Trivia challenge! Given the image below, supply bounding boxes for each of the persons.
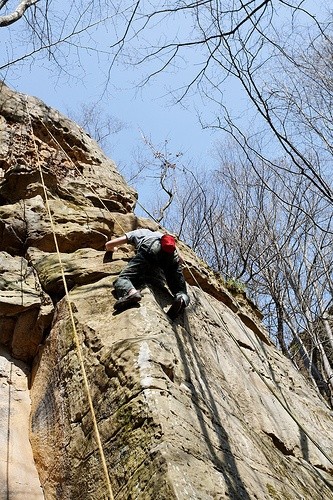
[105,228,190,321]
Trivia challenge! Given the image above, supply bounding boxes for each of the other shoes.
[114,292,141,309]
[167,297,183,320]
